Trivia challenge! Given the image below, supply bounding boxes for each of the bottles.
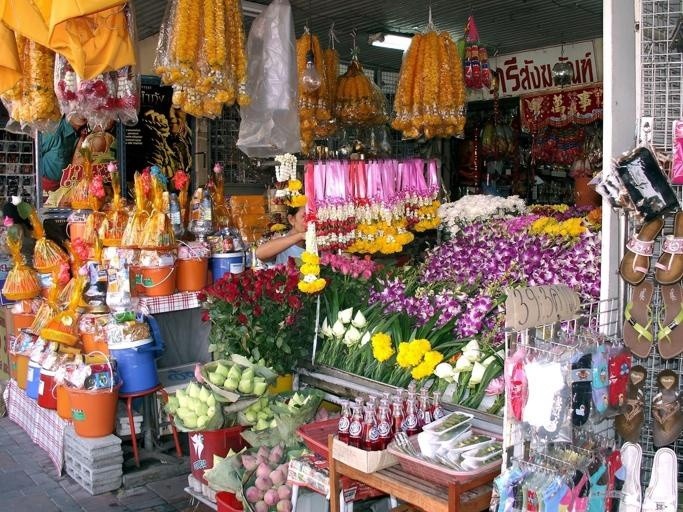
[171,194,181,235]
[337,382,442,451]
[203,191,213,234]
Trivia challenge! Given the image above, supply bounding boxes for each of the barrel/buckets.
[8,333,22,379]
[15,352,30,390]
[140,266,177,296]
[25,360,41,400]
[11,312,35,335]
[127,265,145,294]
[82,334,108,357]
[37,368,57,410]
[63,350,123,438]
[212,252,244,283]
[177,257,209,291]
[51,382,71,420]
[65,222,88,242]
[106,337,161,393]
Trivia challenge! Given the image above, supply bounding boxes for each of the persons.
[255,207,307,265]
[142,105,192,180]
[41,113,86,206]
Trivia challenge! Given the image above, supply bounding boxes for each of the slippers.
[612,363,648,445]
[654,209,683,287]
[639,447,679,512]
[616,441,644,512]
[621,280,655,359]
[649,368,683,448]
[656,283,683,362]
[618,216,666,286]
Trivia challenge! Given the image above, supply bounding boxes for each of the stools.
[118,385,183,468]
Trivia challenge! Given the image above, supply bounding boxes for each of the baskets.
[39,328,78,347]
[386,425,504,488]
[32,253,71,274]
[2,286,41,301]
[294,410,385,503]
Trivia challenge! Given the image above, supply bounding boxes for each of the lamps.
[300,0,322,91]
[552,42,574,86]
[368,32,414,51]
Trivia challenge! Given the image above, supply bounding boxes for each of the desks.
[116,294,202,316]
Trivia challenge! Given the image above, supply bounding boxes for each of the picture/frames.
[33,119,127,219]
